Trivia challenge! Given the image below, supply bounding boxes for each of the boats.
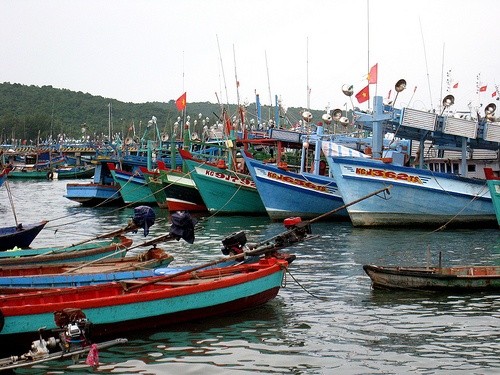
[363,239,499,298]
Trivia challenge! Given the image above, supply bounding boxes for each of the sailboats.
[0,0,500,375]
